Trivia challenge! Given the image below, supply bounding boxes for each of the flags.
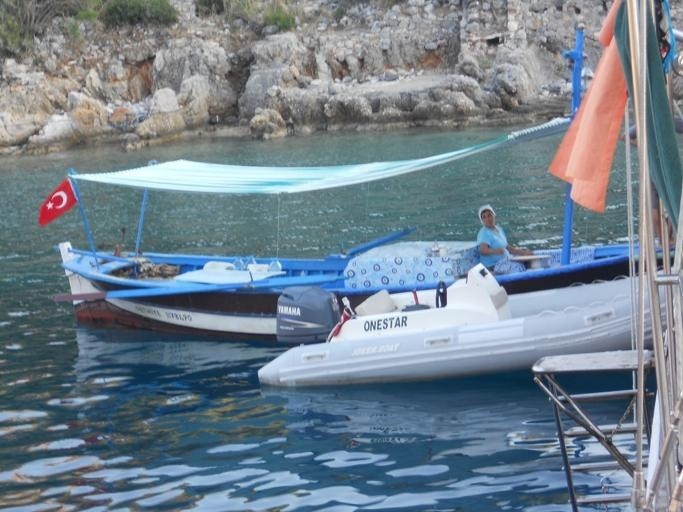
[38,176,78,228]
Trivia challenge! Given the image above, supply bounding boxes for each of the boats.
[57,23,680,342]
[257,262,676,392]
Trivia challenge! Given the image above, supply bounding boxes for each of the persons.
[475,204,533,274]
[617,103,676,247]
